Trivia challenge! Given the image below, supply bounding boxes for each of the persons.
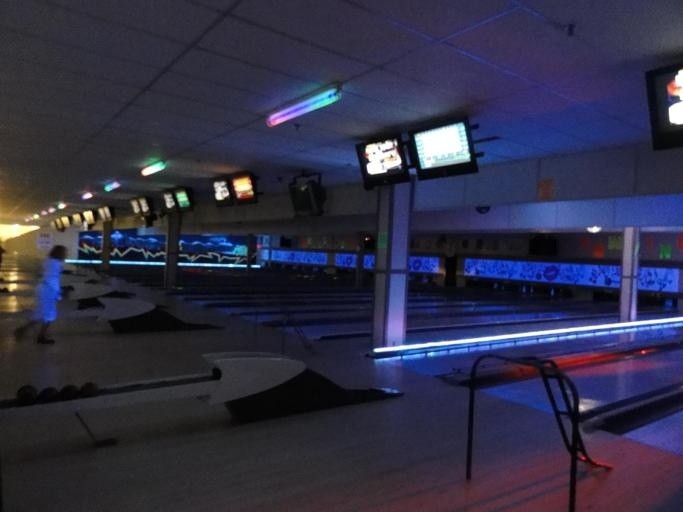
[14,245,65,344]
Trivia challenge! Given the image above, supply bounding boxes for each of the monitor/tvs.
[288,180,327,215]
[355,132,411,190]
[49,170,259,231]
[408,116,479,181]
[645,62,683,151]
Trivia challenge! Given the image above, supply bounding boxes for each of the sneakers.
[14,327,56,345]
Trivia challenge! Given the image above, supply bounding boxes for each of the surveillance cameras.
[475,205,491,214]
